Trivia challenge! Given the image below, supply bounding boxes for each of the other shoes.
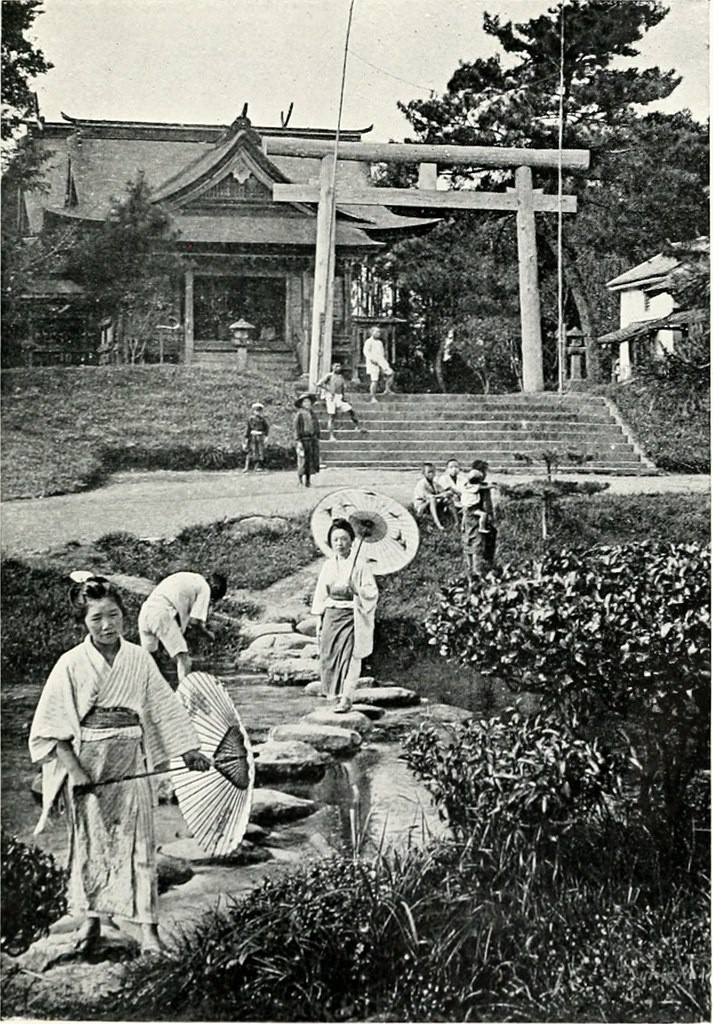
[71,926,101,953]
[333,704,352,713]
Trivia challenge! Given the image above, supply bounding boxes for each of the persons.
[363,325,396,402]
[460,458,501,572]
[316,359,367,442]
[134,568,228,684]
[439,457,469,508]
[292,393,321,487]
[309,515,380,714]
[25,574,212,955]
[241,403,268,472]
[411,462,460,530]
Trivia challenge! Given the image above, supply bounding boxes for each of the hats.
[294,393,317,408]
[252,403,264,410]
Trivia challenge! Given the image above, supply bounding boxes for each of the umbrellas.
[71,669,260,855]
[309,486,421,585]
